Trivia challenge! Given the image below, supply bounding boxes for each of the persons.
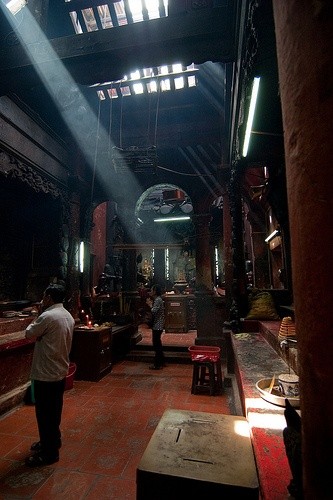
[146,285,165,370]
[24,283,76,466]
[59,248,67,280]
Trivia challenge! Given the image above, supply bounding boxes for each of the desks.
[136,409,259,500]
[112,323,132,335]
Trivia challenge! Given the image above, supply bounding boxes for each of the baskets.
[64,363,76,390]
[188,345,221,363]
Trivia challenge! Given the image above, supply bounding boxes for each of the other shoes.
[31,442,61,451]
[160,362,168,367]
[25,451,59,466]
[150,366,160,370]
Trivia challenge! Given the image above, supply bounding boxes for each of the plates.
[18,314,29,317]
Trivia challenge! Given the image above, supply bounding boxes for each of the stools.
[191,361,223,395]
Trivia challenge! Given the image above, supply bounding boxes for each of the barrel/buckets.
[64,362,76,389]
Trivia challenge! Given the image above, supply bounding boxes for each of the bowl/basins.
[2,311,15,318]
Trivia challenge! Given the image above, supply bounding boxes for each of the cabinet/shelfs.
[72,325,112,381]
